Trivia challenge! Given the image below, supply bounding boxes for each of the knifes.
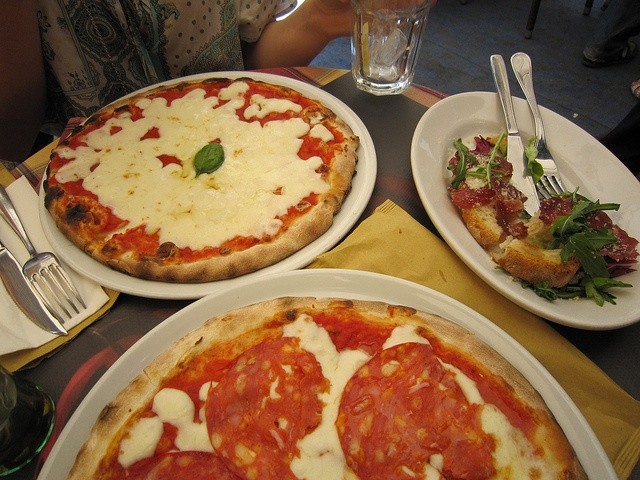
[489,53,541,216]
[0,241,71,337]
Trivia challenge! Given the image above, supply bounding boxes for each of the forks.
[0,183,87,325]
[510,51,569,200]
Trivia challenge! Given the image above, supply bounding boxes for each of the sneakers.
[580,41,636,67]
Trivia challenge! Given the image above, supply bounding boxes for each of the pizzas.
[67,296,582,478]
[43,76,359,282]
[459,139,639,288]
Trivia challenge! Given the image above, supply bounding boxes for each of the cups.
[351,0,432,98]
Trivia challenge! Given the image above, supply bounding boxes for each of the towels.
[308,198,640,480]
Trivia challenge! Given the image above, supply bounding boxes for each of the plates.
[35,268,618,480]
[410,91,640,331]
[38,70,378,302]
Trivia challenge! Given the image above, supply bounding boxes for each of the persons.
[0,0,436,163]
